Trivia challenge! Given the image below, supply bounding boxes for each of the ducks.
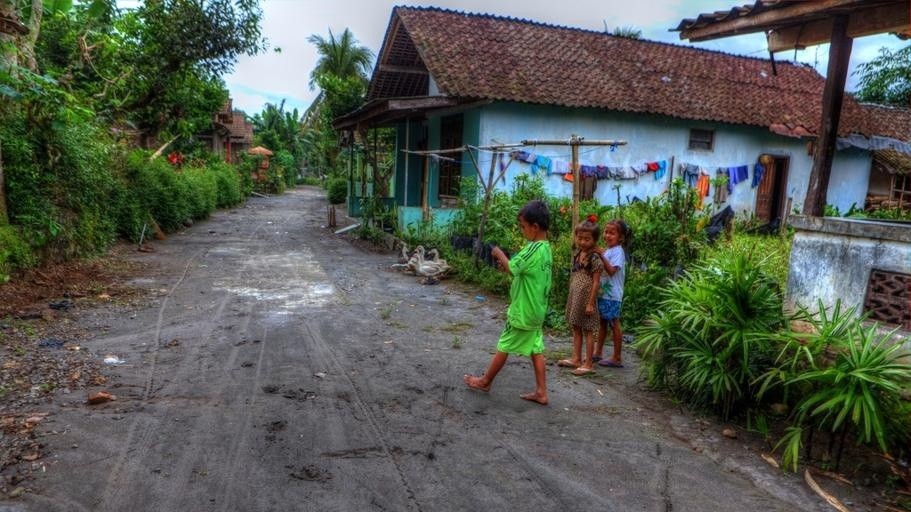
[392,244,453,282]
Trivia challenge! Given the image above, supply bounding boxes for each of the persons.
[584,219,628,368]
[557,213,604,376]
[464,199,554,405]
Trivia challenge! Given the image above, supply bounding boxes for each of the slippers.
[558,359,581,368]
[571,367,597,376]
[598,359,624,368]
[583,355,604,363]
